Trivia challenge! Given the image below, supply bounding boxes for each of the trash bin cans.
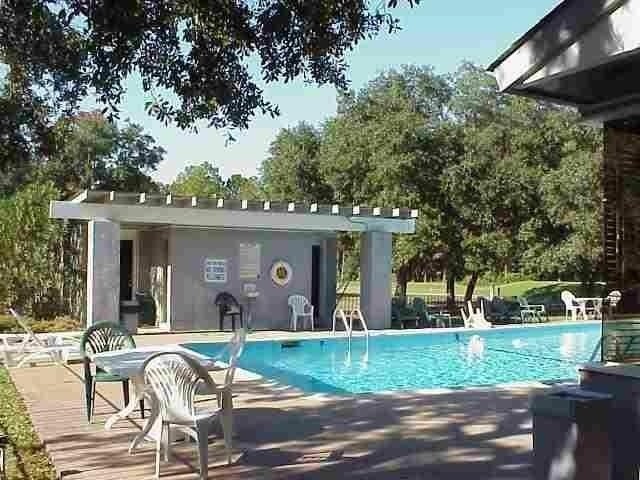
[120,299,141,335]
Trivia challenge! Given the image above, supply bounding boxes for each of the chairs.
[560,289,623,320]
[0,308,76,365]
[81,319,250,480]
[215,289,245,331]
[286,293,318,333]
